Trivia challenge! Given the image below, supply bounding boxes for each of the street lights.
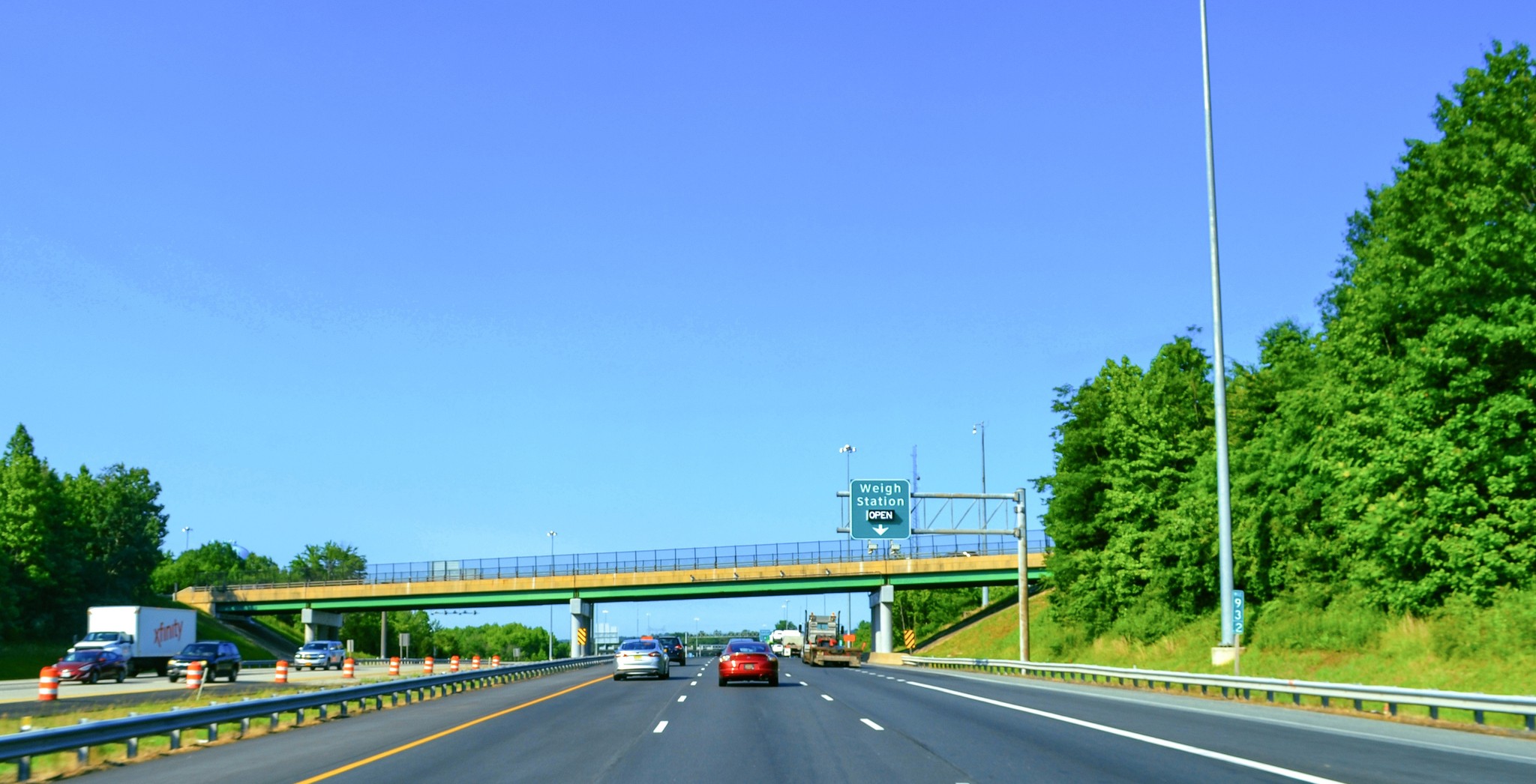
[546,531,558,661]
[972,419,988,555]
[838,444,857,492]
[183,526,191,551]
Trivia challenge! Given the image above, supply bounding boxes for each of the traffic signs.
[849,479,911,541]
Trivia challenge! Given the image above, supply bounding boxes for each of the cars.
[51,648,128,685]
[611,639,671,681]
[715,641,779,688]
[722,638,754,654]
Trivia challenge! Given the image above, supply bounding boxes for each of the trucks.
[767,630,803,657]
[71,605,197,679]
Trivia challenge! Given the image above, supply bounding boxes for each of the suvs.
[655,635,687,666]
[166,640,241,684]
[295,640,346,671]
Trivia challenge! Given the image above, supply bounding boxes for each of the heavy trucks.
[798,612,864,669]
